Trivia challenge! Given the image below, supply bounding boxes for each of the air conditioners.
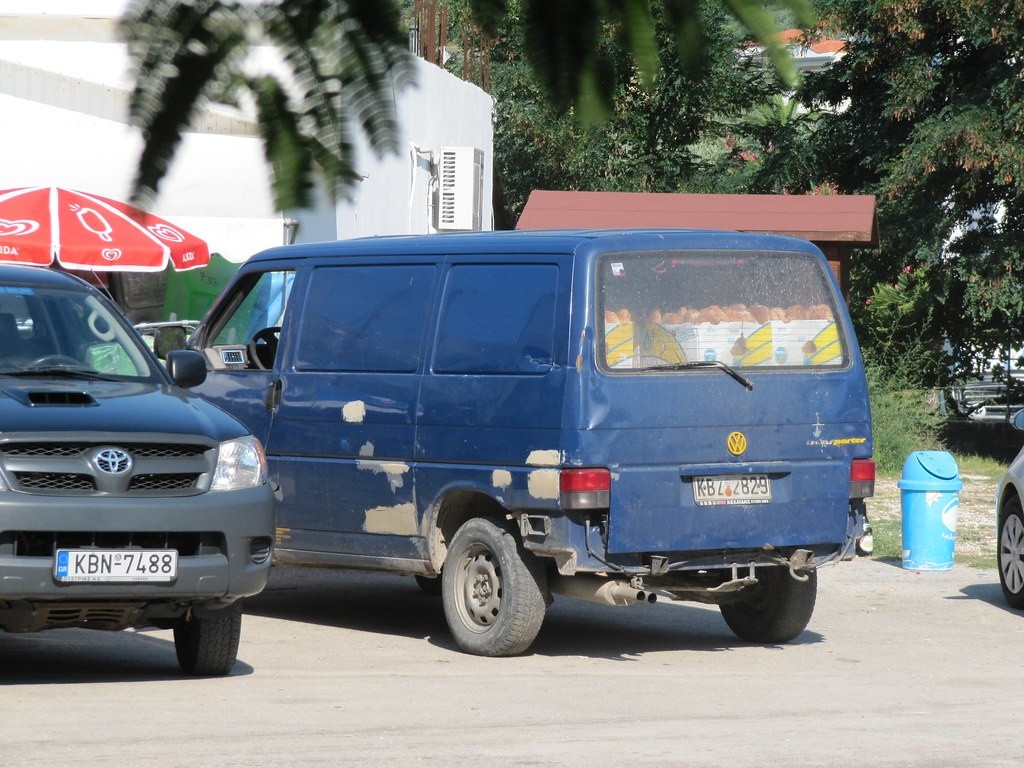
[440,145,484,231]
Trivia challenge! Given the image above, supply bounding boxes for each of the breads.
[604,304,833,324]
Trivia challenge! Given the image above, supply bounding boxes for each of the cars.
[0,260,277,678]
[996,406,1024,610]
[927,341,1023,455]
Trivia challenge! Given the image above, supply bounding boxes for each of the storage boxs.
[640,321,742,367]
[731,321,842,366]
[605,322,636,368]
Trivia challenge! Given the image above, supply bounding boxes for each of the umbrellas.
[0,186,209,272]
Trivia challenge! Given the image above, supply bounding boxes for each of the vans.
[155,226,874,658]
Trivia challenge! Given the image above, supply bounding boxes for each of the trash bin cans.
[898,449,962,573]
